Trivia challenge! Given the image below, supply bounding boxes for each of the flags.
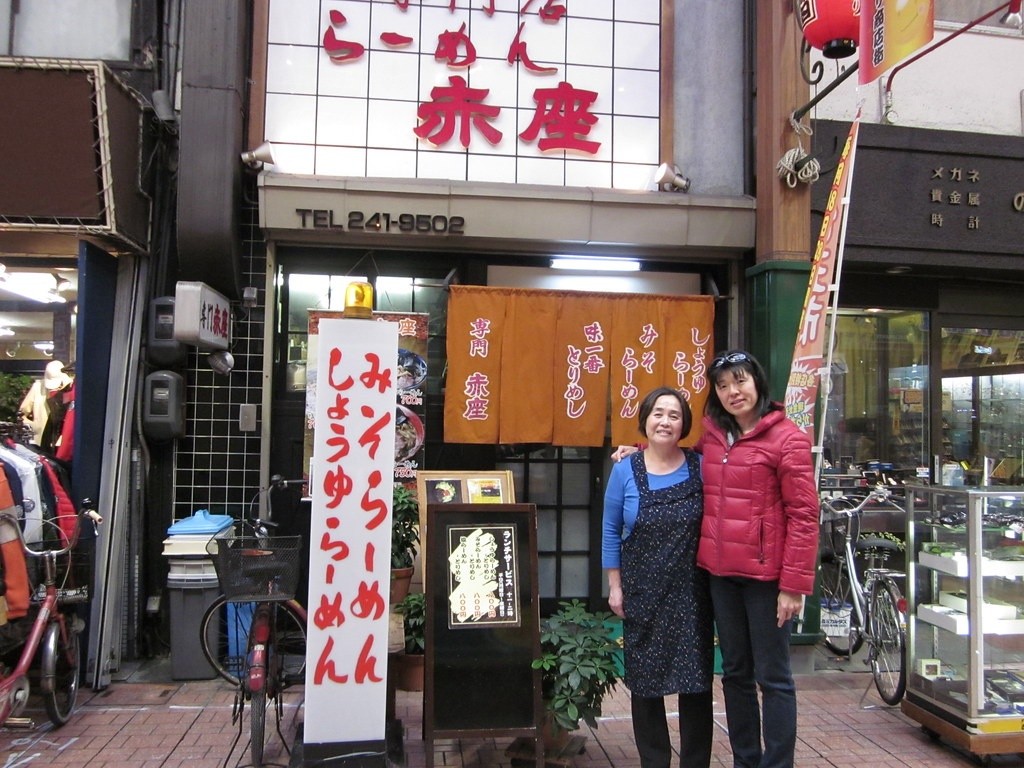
[859,0,934,85]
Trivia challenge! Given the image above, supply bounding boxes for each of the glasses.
[711,353,752,369]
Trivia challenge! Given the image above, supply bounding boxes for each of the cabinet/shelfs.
[903,483,1024,767]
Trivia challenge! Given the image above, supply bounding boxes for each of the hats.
[44,360,65,389]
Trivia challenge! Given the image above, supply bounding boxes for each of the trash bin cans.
[225,576,279,675]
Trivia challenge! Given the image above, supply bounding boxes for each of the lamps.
[240,140,276,169]
[654,162,692,194]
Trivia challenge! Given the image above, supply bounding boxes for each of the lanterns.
[795,0,860,58]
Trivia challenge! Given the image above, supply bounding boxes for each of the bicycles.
[818,482,928,711]
[0,494,105,740]
[197,471,309,768]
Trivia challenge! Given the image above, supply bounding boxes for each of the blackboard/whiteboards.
[425,503,547,739]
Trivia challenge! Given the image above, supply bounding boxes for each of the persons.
[601,386,714,767]
[610,349,819,767]
[18,360,73,456]
